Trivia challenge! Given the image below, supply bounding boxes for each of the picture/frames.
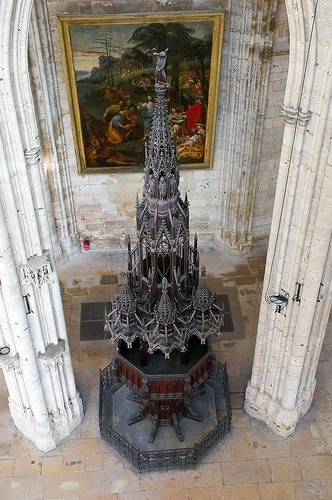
[56,10,226,174]
